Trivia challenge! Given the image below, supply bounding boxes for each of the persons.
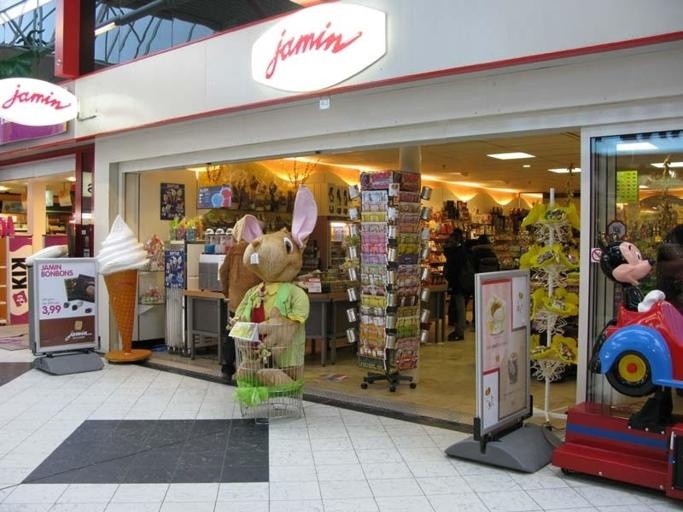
[218,215,268,382]
[439,228,501,341]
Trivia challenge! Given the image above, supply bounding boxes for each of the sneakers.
[448,331,464,341]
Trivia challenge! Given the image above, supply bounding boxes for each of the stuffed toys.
[226,183,318,379]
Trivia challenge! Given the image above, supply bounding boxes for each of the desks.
[332,284,448,363]
[184,289,330,366]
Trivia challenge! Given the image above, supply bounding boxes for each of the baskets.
[232,321,304,421]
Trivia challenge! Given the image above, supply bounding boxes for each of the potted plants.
[226,175,289,212]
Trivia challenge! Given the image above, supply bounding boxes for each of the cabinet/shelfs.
[318,214,352,269]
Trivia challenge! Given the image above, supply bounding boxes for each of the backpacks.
[458,257,474,289]
[479,257,497,272]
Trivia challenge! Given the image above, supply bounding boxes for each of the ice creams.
[96,214,150,353]
